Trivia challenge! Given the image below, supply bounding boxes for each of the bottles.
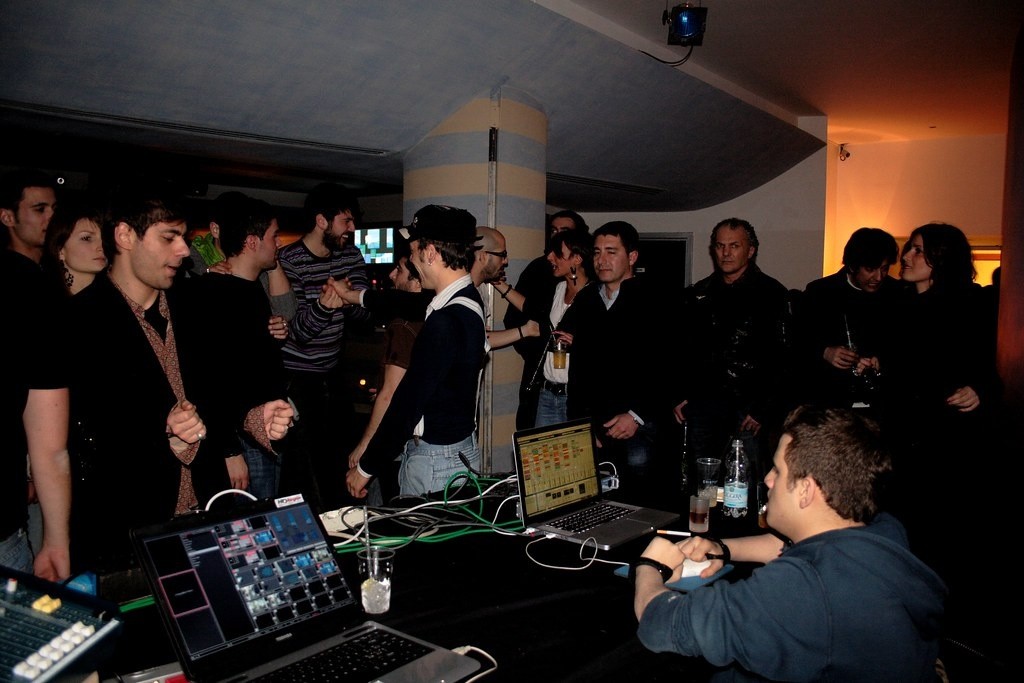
[553,342,568,369]
[845,330,865,376]
[724,439,748,517]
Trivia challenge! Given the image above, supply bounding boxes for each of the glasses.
[486,250,508,259]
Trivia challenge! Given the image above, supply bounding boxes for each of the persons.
[469,209,676,494]
[634,404,956,683]
[676,218,794,451]
[275,181,370,508]
[345,204,490,501]
[794,223,1005,444]
[165,190,295,511]
[0,169,206,581]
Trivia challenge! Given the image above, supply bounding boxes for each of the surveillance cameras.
[841,148,850,158]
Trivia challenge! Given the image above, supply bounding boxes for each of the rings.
[197,433,204,440]
[207,267,210,272]
[282,424,289,434]
[282,322,287,328]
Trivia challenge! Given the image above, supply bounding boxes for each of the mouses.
[682,558,711,578]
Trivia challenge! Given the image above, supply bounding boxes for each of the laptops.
[128,489,482,683]
[512,417,680,550]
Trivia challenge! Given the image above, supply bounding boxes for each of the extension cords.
[317,504,365,531]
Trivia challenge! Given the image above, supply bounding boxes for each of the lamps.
[638,0,707,66]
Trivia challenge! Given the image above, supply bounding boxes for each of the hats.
[393,205,484,245]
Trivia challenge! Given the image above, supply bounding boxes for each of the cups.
[690,494,709,532]
[696,457,721,507]
[757,482,771,528]
[356,548,394,613]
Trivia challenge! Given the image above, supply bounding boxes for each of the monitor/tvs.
[355,228,394,264]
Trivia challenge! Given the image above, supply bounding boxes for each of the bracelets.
[634,556,672,583]
[633,417,638,425]
[518,326,524,339]
[501,284,512,298]
